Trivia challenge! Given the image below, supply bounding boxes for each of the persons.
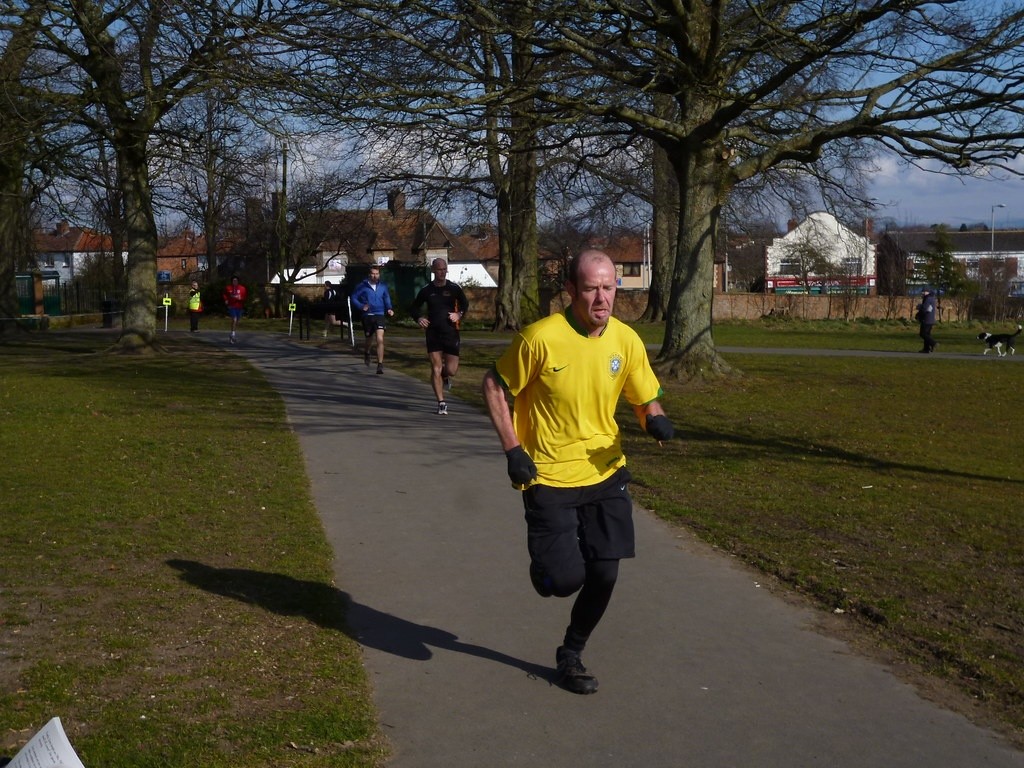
[188,280,203,334]
[319,281,355,340]
[483,247,674,694]
[915,286,938,354]
[410,257,469,417]
[224,277,247,345]
[351,266,395,374]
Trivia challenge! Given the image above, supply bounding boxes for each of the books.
[6,716,90,768]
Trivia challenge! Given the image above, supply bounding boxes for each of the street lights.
[991,204,1007,257]
[865,196,878,295]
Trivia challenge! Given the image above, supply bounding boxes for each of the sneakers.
[438,404,448,415]
[556,644,600,693]
[529,563,552,597]
[441,366,452,390]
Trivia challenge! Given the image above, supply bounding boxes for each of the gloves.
[505,445,538,486]
[645,412,673,442]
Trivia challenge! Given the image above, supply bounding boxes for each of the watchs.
[459,310,464,314]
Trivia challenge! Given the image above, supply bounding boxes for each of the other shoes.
[919,350,930,354]
[229,333,236,344]
[192,330,201,333]
[930,341,936,352]
[376,365,384,374]
[365,352,372,364]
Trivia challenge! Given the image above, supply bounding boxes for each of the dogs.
[977,324,1022,358]
